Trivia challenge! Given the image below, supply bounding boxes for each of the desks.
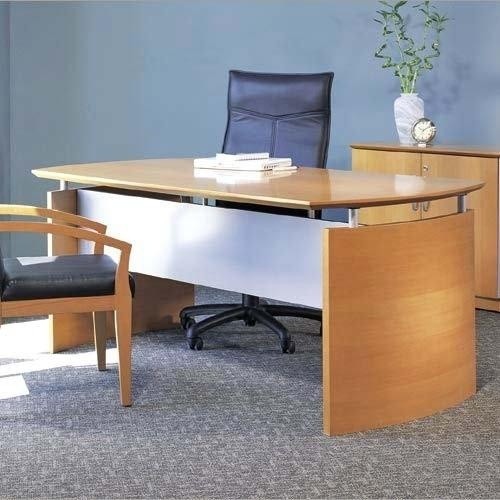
[28,155,491,436]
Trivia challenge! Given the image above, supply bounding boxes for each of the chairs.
[0,203,136,409]
[177,69,336,352]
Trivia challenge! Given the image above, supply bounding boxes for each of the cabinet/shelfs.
[349,142,500,313]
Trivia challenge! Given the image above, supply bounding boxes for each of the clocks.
[409,117,439,149]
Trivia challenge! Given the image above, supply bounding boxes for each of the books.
[193,157,293,171]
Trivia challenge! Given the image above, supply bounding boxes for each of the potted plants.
[370,1,457,146]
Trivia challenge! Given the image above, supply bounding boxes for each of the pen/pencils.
[274,166,299,169]
